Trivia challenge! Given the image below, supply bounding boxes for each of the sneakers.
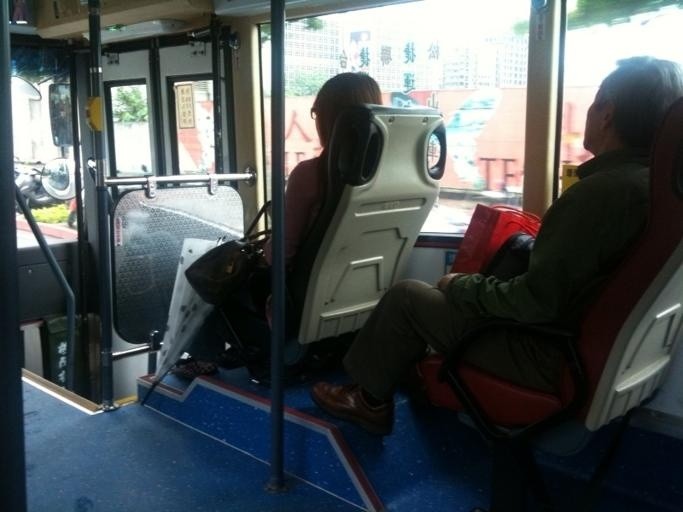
[158,350,218,378]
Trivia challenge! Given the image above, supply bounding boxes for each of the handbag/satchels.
[182,199,283,310]
[445,202,545,283]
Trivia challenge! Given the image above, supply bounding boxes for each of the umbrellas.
[139,200,272,407]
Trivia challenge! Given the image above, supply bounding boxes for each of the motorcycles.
[13,156,67,212]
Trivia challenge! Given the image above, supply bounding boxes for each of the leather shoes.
[304,379,394,438]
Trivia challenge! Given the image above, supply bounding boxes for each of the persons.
[308,55,683,442]
[169,71,381,382]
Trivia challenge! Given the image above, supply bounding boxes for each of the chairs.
[418,105,683,482]
[172,109,449,385]
